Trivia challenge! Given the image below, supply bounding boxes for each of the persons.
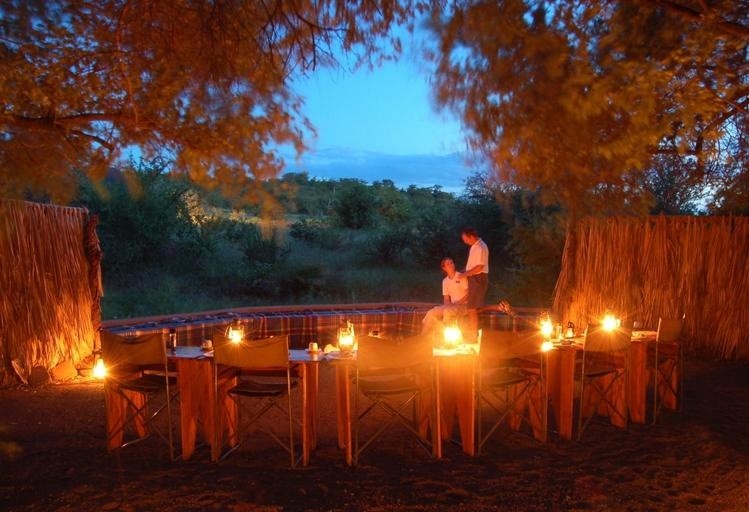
[458,228,519,344]
[421,256,469,336]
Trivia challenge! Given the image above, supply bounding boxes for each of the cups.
[555,325,563,339]
[201,339,212,351]
[309,342,318,350]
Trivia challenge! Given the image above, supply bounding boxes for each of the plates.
[204,349,215,360]
[304,348,321,353]
[327,350,356,360]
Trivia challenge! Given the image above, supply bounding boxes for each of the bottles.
[168,328,176,350]
[565,320,576,339]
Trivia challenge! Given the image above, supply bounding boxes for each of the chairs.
[454,333,553,462]
[608,315,684,432]
[100,326,199,462]
[320,340,448,463]
[516,326,634,451]
[197,330,323,466]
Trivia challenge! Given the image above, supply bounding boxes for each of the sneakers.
[498,299,519,321]
